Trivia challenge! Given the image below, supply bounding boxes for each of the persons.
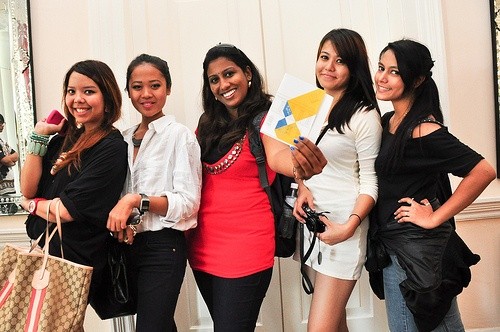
[363,36,496,332]
[0,114,18,180]
[18,60,128,307]
[290,28,382,332]
[106,54,203,332]
[186,44,327,332]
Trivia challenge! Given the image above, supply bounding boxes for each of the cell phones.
[46,110,67,135]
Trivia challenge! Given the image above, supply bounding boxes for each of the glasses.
[216,44,240,55]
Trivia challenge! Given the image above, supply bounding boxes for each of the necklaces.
[133,137,141,147]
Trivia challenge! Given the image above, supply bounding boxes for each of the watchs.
[139,193,150,213]
[27,198,46,215]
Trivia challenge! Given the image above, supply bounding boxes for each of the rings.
[410,198,414,204]
[405,212,408,217]
[425,203,430,206]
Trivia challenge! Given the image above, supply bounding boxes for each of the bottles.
[277,182,300,239]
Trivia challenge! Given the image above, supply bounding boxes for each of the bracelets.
[31,132,49,144]
[350,214,362,224]
[27,141,48,157]
[293,167,297,178]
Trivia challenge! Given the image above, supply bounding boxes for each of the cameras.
[299,204,328,233]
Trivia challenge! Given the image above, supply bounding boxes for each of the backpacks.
[249,110,299,258]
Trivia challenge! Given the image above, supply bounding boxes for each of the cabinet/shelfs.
[127,256,388,332]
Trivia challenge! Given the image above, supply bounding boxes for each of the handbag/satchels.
[89,231,137,320]
[0,200,93,332]
